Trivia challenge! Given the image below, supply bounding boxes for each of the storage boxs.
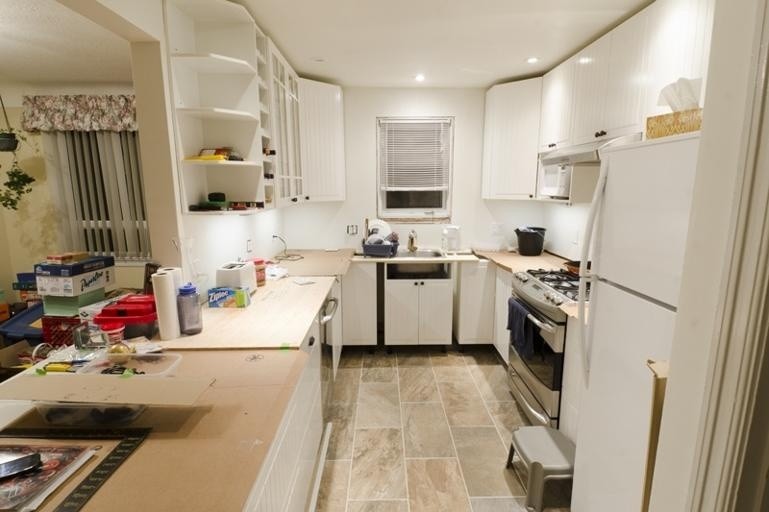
[0,255,159,383]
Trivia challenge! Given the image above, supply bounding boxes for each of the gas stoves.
[526,268,591,301]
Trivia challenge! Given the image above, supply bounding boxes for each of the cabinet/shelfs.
[162,0,347,216]
[243,263,514,512]
[481,0,715,205]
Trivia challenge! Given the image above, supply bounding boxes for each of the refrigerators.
[568,131,704,511]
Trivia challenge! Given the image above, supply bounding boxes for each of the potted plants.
[0,128,40,211]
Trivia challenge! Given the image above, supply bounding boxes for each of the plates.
[362,218,392,245]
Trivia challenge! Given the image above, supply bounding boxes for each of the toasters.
[216,261,257,295]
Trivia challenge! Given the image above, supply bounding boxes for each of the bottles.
[408,230,417,251]
[441,229,449,255]
[0,288,9,324]
[254,258,266,287]
[176,283,203,336]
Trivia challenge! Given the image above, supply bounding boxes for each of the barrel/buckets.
[513,226,546,256]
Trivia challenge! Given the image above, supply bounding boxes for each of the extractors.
[542,131,643,167]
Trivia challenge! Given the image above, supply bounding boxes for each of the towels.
[507,297,535,360]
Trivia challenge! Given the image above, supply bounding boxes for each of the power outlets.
[272,233,278,241]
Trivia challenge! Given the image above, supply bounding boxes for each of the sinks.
[396,249,442,274]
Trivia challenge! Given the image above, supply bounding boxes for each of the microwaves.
[535,152,572,199]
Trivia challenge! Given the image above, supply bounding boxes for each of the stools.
[505,426,576,512]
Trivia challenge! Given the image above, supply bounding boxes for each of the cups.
[384,231,400,242]
[100,321,126,345]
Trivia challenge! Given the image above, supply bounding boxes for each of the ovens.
[506,271,568,430]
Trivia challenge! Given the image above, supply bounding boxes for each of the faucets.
[407,230,418,254]
